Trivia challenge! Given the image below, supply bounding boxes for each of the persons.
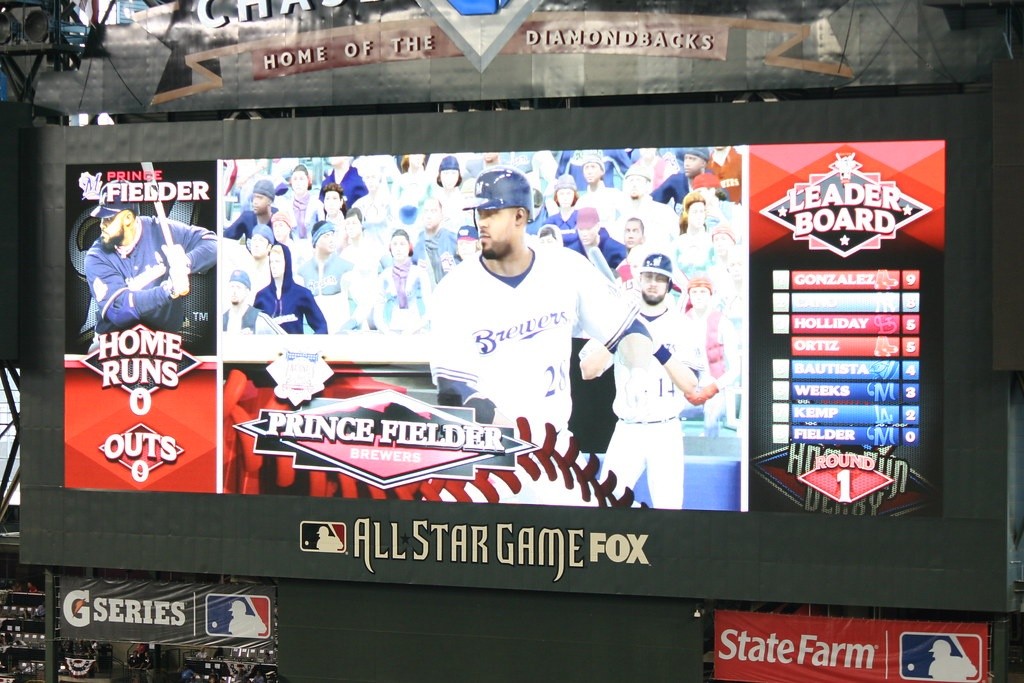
[578,223,715,511]
[0,577,99,673]
[219,157,742,429]
[126,644,273,683]
[80,181,217,352]
[428,166,643,462]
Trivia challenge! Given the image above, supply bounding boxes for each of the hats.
[271,211,293,229]
[438,156,459,170]
[710,221,737,243]
[687,277,712,295]
[252,223,275,245]
[90,176,139,219]
[574,206,599,229]
[623,167,653,182]
[583,154,605,172]
[685,147,709,160]
[554,173,577,192]
[683,192,706,205]
[458,226,479,241]
[463,165,534,223]
[693,173,720,189]
[229,270,251,289]
[639,254,672,281]
[252,180,275,202]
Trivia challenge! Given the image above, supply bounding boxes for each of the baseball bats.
[137,161,193,296]
